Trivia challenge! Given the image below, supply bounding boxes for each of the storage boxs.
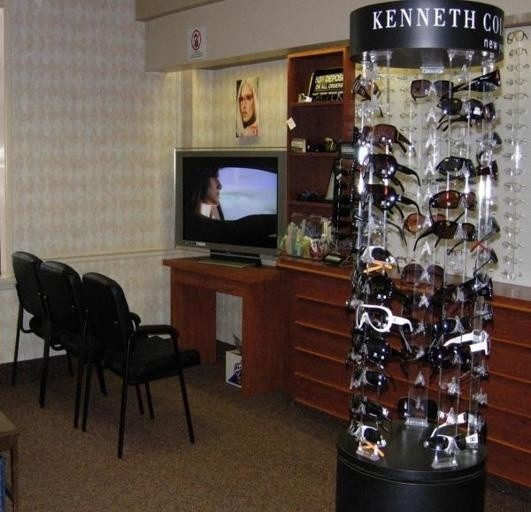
[224,348,243,390]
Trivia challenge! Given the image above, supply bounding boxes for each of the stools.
[0,408,21,512]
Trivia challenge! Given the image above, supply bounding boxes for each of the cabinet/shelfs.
[286,45,352,266]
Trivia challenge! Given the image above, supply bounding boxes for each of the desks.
[161,255,287,400]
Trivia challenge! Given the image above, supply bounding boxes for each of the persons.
[183,158,222,220]
[235,76,260,136]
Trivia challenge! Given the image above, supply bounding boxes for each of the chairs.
[80,270,204,462]
[10,250,107,409]
[38,258,146,430]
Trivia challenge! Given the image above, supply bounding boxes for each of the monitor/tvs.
[174,147,287,268]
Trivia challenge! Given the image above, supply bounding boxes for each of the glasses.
[352,75,381,101]
[411,69,501,131]
[346,125,501,457]
[502,30,528,281]
